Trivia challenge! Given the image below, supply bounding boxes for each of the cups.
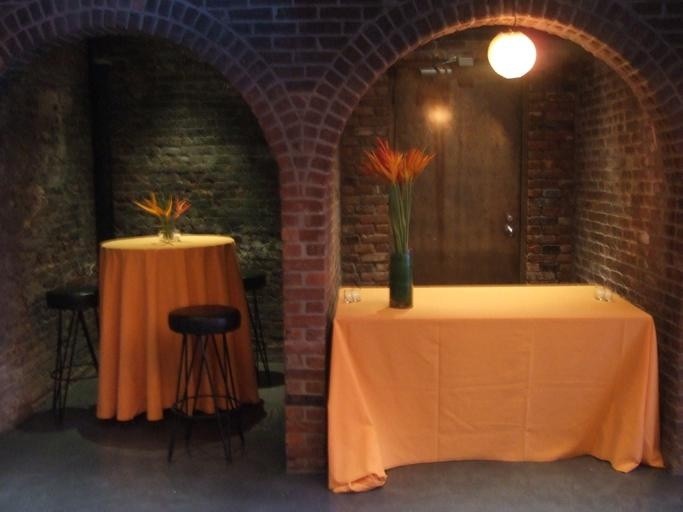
[593,282,613,303]
[344,286,361,305]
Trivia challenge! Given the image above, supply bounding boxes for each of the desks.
[95,233,267,429]
[327,283,665,494]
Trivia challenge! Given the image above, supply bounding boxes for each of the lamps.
[487,25,536,79]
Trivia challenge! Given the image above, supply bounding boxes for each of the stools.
[167,303,246,464]
[45,285,100,424]
[241,269,275,387]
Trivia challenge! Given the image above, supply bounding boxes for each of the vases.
[389,252,413,308]
[158,225,181,243]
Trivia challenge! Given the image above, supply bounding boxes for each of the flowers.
[133,191,192,226]
[364,136,437,254]
